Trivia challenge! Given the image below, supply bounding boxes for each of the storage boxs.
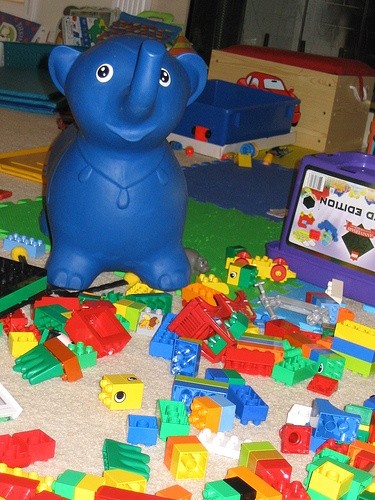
[205,45,375,155]
